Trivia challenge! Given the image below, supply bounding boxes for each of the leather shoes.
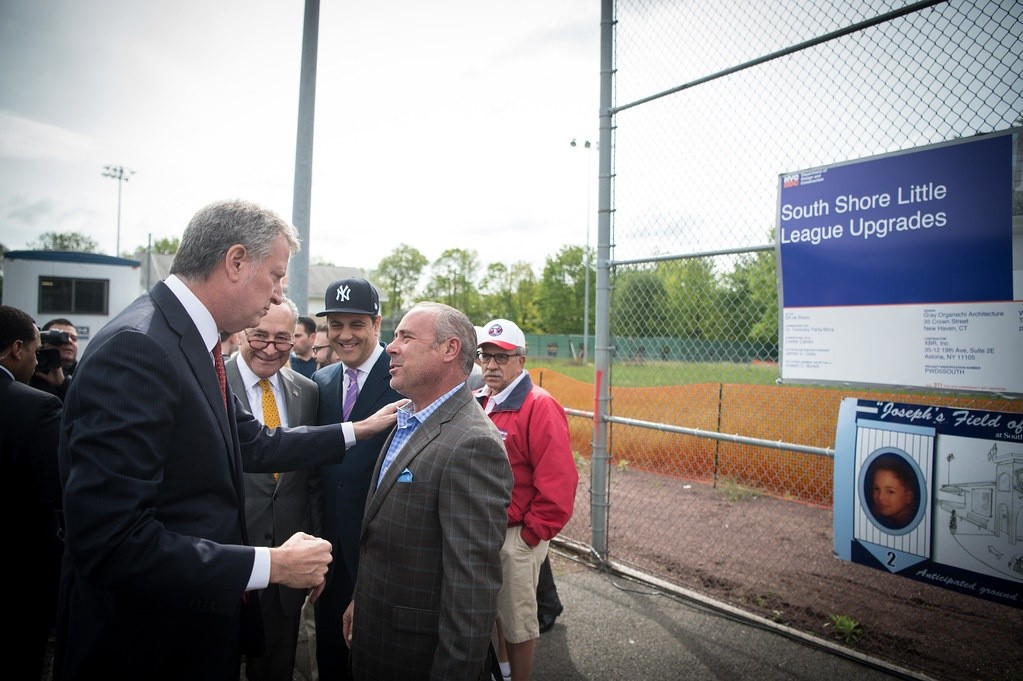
[538,606,565,632]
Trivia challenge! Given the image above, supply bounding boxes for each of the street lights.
[102,163,138,258]
[570,135,596,364]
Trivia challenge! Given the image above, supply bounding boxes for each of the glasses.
[477,352,522,364]
[311,344,334,355]
[243,331,294,352]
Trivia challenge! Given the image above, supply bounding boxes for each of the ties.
[343,367,359,422]
[212,335,228,413]
[258,379,282,481]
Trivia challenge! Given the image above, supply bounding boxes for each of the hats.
[476,319,525,352]
[317,278,379,315]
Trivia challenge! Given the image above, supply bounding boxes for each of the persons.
[26,319,77,401]
[0,304,63,681]
[473,319,579,681]
[342,302,513,681]
[309,278,408,681]
[55,197,335,680]
[221,293,320,679]
[870,457,918,529]
[213,316,340,380]
